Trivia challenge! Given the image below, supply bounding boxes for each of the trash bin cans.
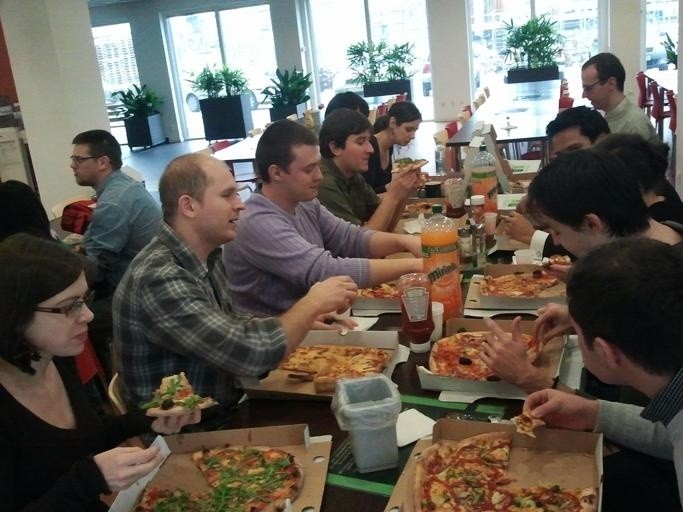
[336,375,401,473]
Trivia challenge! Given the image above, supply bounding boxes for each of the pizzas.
[134,444,303,512]
[546,254,572,265]
[413,431,600,512]
[510,409,546,438]
[428,330,544,383]
[481,269,557,298]
[391,157,429,172]
[145,374,215,420]
[357,282,401,300]
[280,345,390,382]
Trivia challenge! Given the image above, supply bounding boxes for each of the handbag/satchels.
[61,200,96,234]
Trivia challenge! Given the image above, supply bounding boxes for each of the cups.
[416,181,440,198]
[430,300,443,344]
[456,227,472,254]
[443,179,466,211]
[485,212,497,236]
[514,250,538,266]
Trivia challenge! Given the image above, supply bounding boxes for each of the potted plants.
[345,39,415,105]
[186,64,254,142]
[499,14,562,81]
[107,84,171,152]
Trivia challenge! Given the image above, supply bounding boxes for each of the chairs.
[75,334,108,401]
[431,81,577,170]
[104,374,145,453]
[200,91,409,189]
[633,68,677,167]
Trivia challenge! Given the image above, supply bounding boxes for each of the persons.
[361,102,422,194]
[325,92,369,117]
[478,54,682,511]
[0,232,202,512]
[222,120,424,318]
[315,107,417,232]
[70,130,164,332]
[111,153,358,448]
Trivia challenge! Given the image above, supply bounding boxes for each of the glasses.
[35,289,96,318]
[70,156,100,162]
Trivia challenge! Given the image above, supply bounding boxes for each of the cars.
[644,41,669,72]
[181,68,279,112]
[103,83,133,120]
[329,63,382,104]
[421,54,480,98]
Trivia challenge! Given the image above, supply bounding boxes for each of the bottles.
[470,145,499,220]
[505,117,510,127]
[434,141,445,162]
[471,225,486,253]
[400,272,436,353]
[469,194,485,225]
[419,204,462,319]
